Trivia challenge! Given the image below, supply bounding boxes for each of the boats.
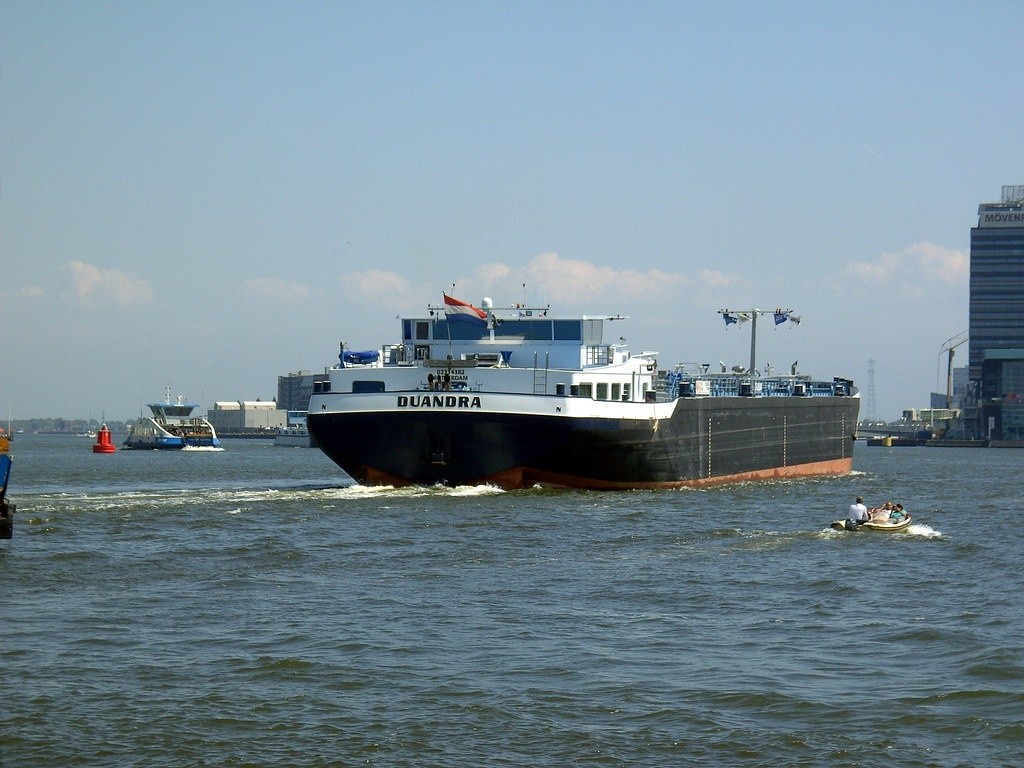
[122,396,221,450]
[16,428,24,435]
[830,505,912,535]
[306,283,860,491]
[274,426,316,448]
[85,431,97,439]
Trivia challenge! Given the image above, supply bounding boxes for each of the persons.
[848,497,868,520]
[881,502,907,525]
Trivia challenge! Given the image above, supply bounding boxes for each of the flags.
[444,294,487,329]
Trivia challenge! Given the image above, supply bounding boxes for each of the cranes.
[938,329,969,409]
